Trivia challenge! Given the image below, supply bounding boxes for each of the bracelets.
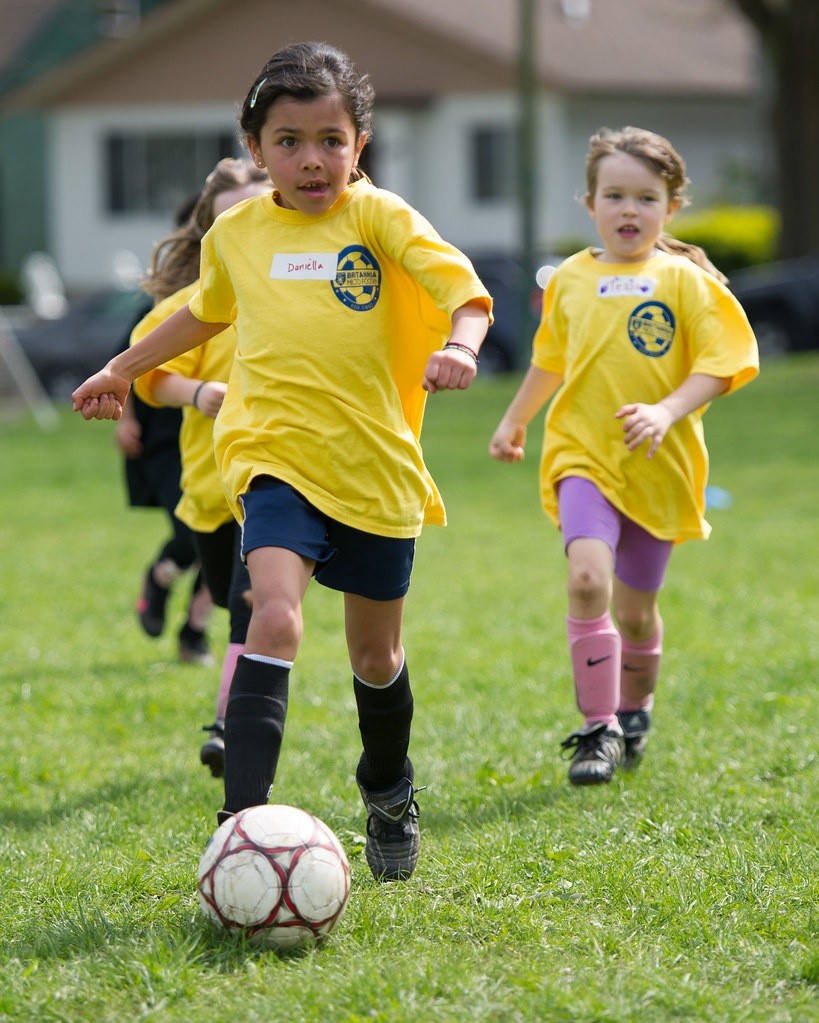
[444,341,481,365]
[193,380,208,410]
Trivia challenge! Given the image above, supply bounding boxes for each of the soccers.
[195,803,355,953]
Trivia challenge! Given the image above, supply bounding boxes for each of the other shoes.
[179,622,216,667]
[200,718,225,777]
[141,568,168,637]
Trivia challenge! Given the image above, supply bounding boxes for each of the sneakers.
[355,750,427,884]
[560,720,626,785]
[616,694,654,755]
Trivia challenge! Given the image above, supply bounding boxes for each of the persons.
[103,154,282,781]
[72,39,492,888]
[492,125,761,787]
